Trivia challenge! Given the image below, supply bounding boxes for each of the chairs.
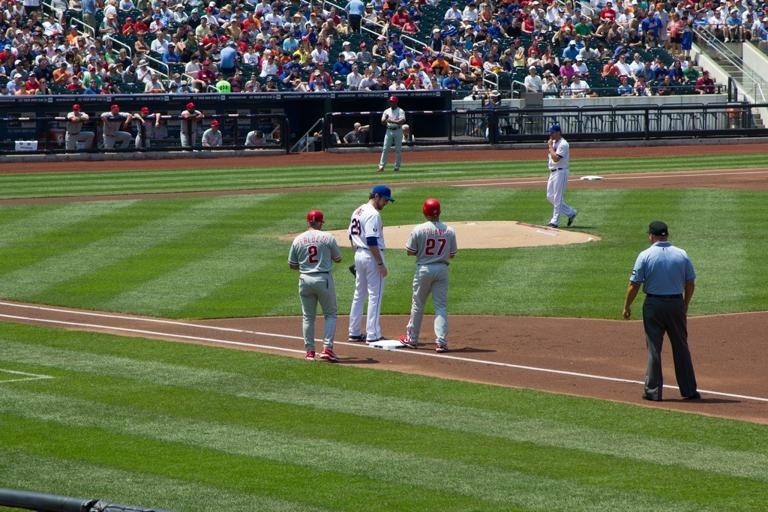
[42,0,696,95]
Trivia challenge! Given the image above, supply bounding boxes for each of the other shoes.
[708,33,760,43]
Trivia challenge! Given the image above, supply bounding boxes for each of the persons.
[1,1,470,154]
[348,185,395,344]
[288,210,343,362]
[379,96,406,171]
[442,1,767,145]
[546,125,577,228]
[399,197,458,352]
[623,221,701,401]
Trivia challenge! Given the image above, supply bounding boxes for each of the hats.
[544,125,560,132]
[646,222,668,235]
[209,120,220,127]
[72,104,196,115]
[369,186,395,201]
[386,96,398,103]
[489,89,501,96]
[0,0,708,90]
[709,1,762,17]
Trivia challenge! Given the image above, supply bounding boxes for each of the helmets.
[423,198,440,217]
[306,210,325,223]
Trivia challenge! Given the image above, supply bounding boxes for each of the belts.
[551,168,561,172]
[388,126,400,130]
[399,335,417,348]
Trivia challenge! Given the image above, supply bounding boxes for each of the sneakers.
[681,391,701,399]
[348,335,366,341]
[567,214,575,226]
[436,343,448,351]
[376,167,384,173]
[546,223,558,228]
[305,351,315,360]
[366,337,385,344]
[319,347,337,362]
[642,392,661,400]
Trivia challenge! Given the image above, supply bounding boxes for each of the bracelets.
[378,263,383,265]
